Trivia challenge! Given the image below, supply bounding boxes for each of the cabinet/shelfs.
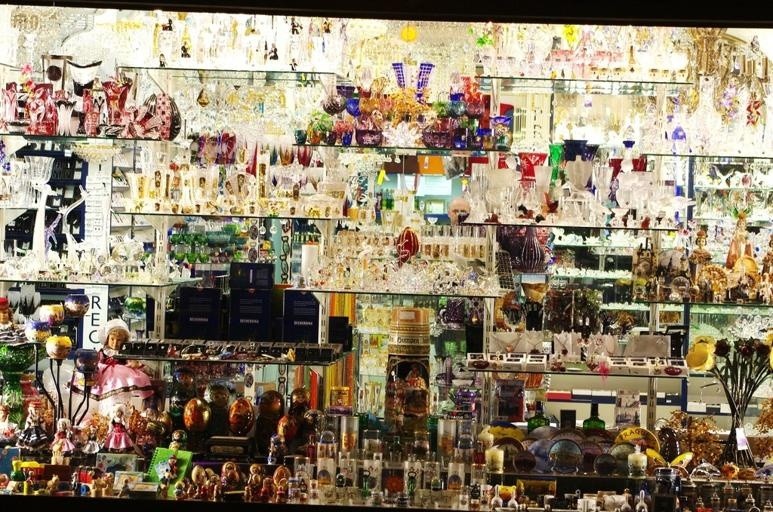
[0,61,773,487]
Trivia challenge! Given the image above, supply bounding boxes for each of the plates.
[486,419,693,476]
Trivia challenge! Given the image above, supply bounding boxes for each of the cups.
[0,9,773,309]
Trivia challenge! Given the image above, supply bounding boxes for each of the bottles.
[288,310,519,510]
[582,400,606,432]
[527,398,549,432]
[520,467,772,511]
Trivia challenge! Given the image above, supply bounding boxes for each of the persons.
[763,233,773,273]
[725,211,752,269]
[689,229,712,266]
[447,199,471,225]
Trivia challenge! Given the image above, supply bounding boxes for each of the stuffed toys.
[15,317,155,468]
